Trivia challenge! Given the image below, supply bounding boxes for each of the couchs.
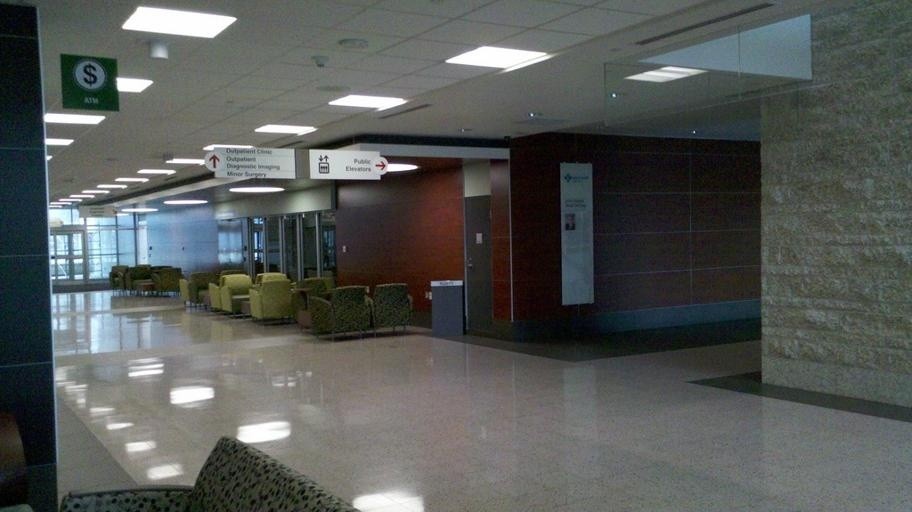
[310,282,416,339]
[107,263,187,298]
[179,271,331,333]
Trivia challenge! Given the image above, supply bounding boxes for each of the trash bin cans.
[430,280,464,337]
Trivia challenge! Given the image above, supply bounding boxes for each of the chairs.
[60,436,362,512]
